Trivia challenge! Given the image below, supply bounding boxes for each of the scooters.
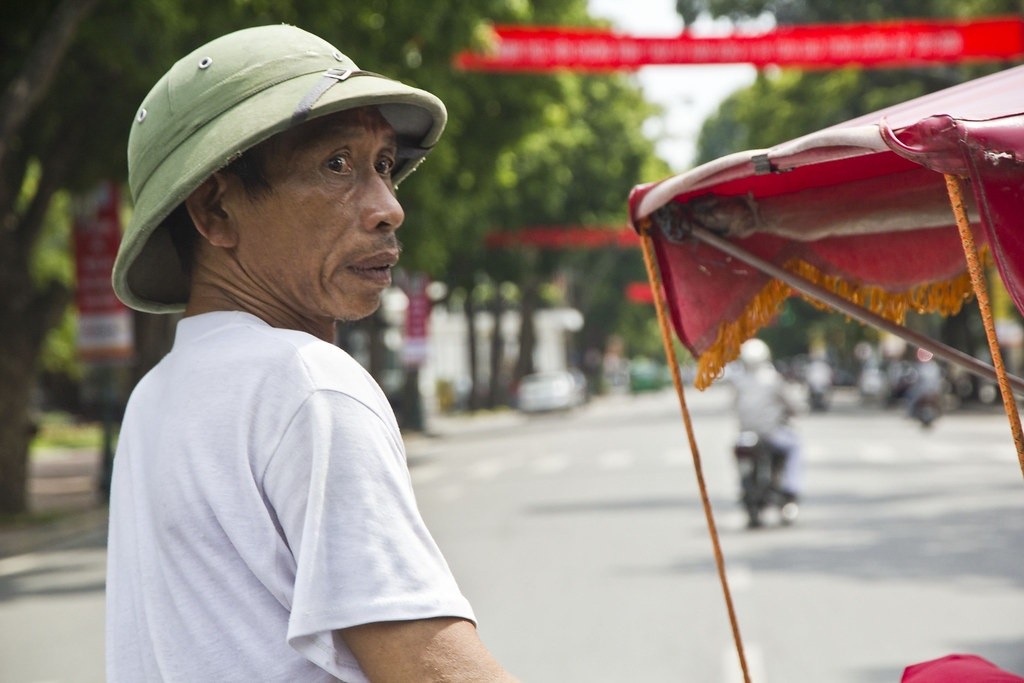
[913,394,944,427]
[735,403,799,527]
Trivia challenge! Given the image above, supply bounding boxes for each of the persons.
[104,24,521,683]
[731,338,801,497]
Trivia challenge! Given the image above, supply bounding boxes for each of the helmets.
[740,338,771,368]
[110,22,444,315]
